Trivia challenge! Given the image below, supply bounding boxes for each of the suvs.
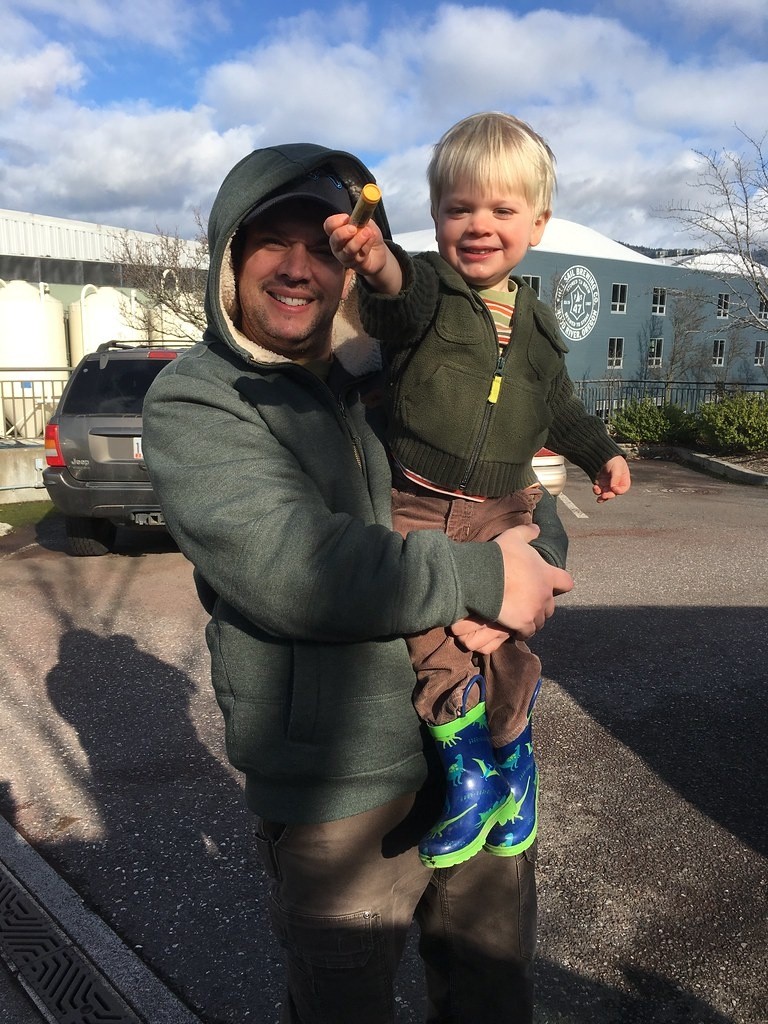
[42,340,192,556]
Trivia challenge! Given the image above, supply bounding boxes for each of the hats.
[240,166,352,227]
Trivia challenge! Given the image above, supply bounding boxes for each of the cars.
[532,447,567,496]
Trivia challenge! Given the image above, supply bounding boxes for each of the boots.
[483,709,539,857]
[421,702,515,869]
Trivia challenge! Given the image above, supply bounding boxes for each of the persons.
[141,143,576,1024]
[323,111,632,870]
